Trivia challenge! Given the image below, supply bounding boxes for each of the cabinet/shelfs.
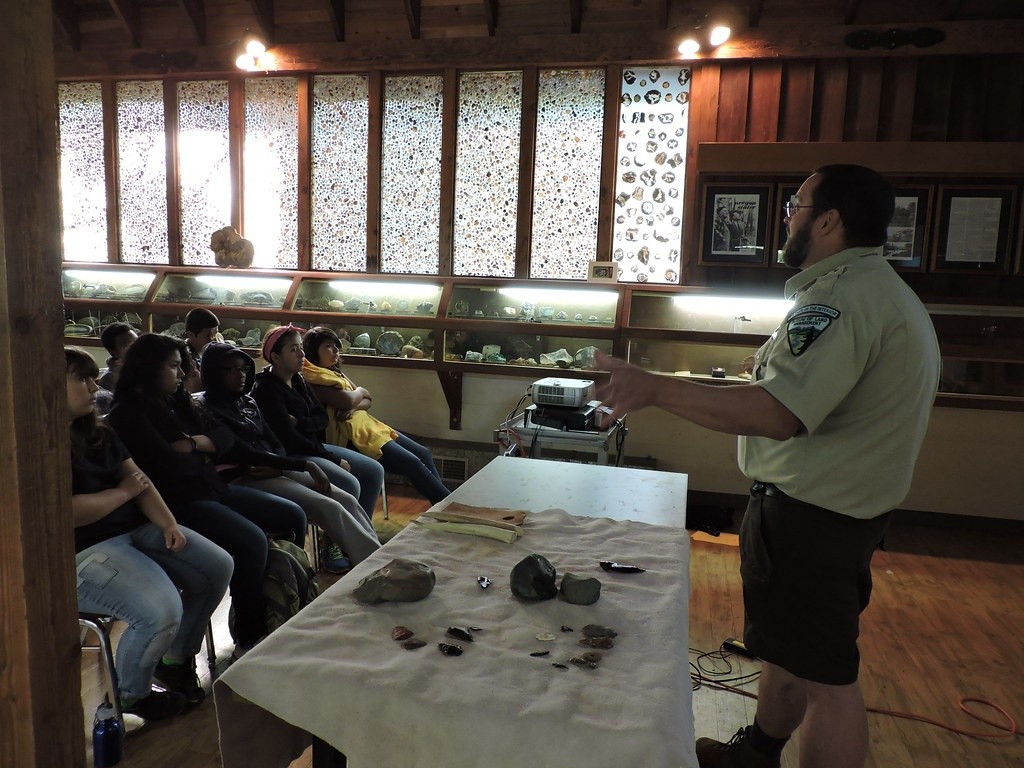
[60,260,1024,537]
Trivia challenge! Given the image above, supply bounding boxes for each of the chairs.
[72,608,214,739]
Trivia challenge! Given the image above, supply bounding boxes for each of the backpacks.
[228,531,321,649]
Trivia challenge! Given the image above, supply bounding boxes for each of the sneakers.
[100,691,187,720]
[151,658,211,704]
[320,538,351,574]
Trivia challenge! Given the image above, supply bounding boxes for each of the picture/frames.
[696,180,774,268]
[587,260,618,284]
[772,183,802,268]
[879,182,937,272]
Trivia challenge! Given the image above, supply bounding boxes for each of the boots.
[697,721,790,768]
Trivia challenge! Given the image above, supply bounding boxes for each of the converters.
[724,637,752,657]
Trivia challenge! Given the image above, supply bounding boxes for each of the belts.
[753,480,792,501]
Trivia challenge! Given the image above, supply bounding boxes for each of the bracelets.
[187,435,198,450]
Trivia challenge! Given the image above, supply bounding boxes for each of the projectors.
[531,377,596,408]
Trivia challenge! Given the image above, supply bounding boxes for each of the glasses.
[225,365,253,373]
[784,201,839,218]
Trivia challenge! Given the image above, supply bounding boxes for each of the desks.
[212,463,690,768]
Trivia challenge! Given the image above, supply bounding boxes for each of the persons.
[62,306,454,721]
[582,162,944,768]
